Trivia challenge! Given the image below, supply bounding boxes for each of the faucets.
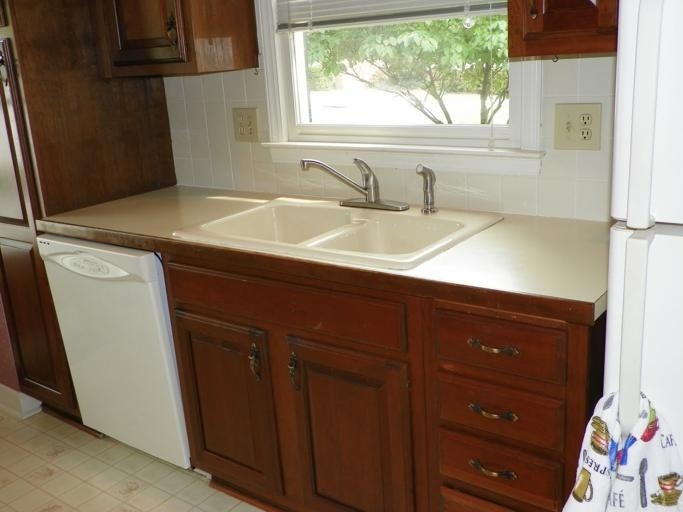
[301,158,409,212]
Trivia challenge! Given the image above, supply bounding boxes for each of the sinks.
[306,208,504,268]
[171,192,359,271]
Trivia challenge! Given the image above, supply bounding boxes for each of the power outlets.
[231,107,261,144]
[554,103,601,152]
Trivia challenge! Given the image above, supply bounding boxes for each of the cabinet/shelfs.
[89,1,258,81]
[160,243,422,512]
[507,0,617,60]
[417,278,589,512]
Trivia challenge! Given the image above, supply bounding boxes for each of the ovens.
[35,233,193,471]
